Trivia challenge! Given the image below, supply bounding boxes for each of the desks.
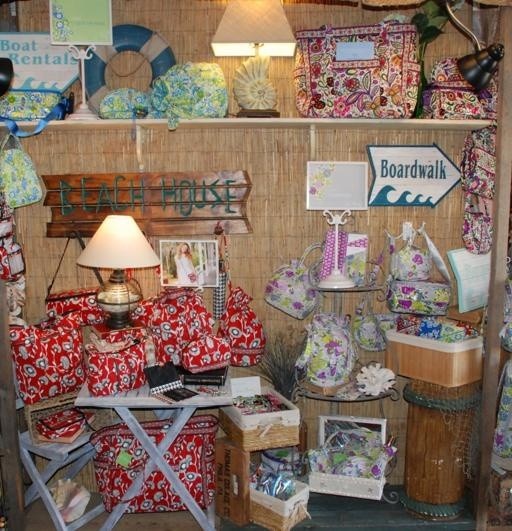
[73,377,233,531]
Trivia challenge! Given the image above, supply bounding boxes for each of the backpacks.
[1,89,63,281]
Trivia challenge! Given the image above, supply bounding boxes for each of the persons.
[173,241,199,284]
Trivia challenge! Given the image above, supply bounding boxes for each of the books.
[157,386,199,404]
[36,419,87,444]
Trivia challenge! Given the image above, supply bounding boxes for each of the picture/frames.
[306,160,368,210]
[318,416,387,446]
[159,240,219,287]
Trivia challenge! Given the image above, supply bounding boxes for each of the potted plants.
[411,0,448,119]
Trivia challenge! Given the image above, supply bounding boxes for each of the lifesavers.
[81,24,175,117]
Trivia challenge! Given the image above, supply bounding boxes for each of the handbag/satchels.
[386,244,452,316]
[488,278,512,477]
[89,415,218,514]
[309,447,397,479]
[430,58,499,254]
[100,62,228,118]
[261,262,386,387]
[10,287,267,406]
[292,13,421,118]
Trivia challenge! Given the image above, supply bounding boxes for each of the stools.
[400,380,482,522]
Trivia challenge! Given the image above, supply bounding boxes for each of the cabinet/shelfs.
[292,242,400,416]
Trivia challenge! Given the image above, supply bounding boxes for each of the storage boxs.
[215,421,308,527]
[217,386,301,452]
[385,328,483,387]
[234,478,310,531]
[308,472,384,500]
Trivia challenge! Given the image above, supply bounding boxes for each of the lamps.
[75,215,161,329]
[211,0,296,118]
[445,0,504,89]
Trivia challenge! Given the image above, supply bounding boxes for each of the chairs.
[16,398,105,531]
